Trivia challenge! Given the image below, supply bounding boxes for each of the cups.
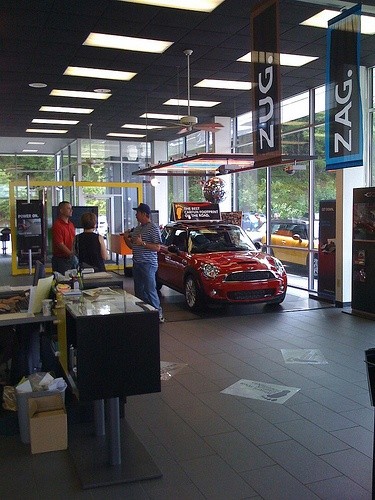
[40,300,52,316]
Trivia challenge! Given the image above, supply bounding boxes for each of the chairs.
[194,235,208,246]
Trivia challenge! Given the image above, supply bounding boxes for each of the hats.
[132,203,151,214]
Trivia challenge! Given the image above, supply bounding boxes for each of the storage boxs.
[28,393,68,454]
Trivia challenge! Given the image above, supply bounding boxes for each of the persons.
[52,200,79,275]
[123,203,165,323]
[71,211,107,273]
[254,213,263,226]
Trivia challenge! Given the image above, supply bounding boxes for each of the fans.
[168,50,224,135]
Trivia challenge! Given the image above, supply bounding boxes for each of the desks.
[0,286,57,374]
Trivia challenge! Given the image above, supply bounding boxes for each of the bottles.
[77,272,84,290]
[74,279,79,289]
[30,368,43,391]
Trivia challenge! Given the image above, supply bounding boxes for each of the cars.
[257,212,319,279]
[154,202,288,314]
[241,211,267,250]
[96,215,107,235]
